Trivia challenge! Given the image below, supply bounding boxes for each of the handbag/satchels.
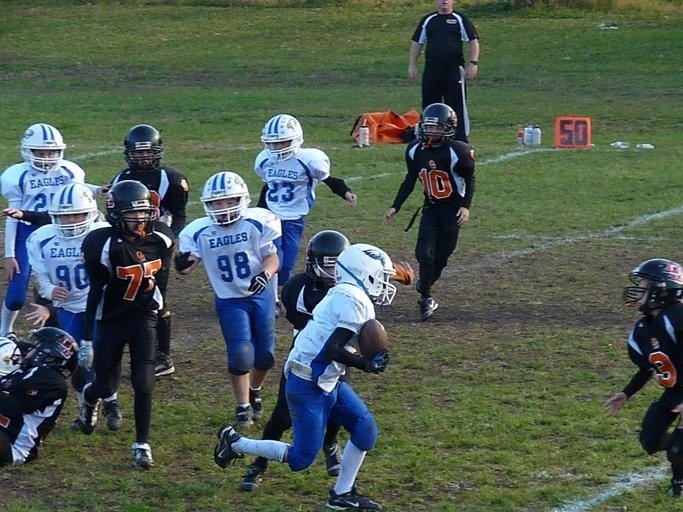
[349,109,420,144]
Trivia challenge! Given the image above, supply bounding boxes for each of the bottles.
[515,124,541,147]
[358,125,369,147]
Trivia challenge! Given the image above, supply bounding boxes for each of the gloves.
[364,348,390,373]
[248,271,272,296]
[392,261,414,285]
[173,250,196,271]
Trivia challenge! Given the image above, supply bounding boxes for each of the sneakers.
[326,484,385,512]
[323,441,342,476]
[420,296,439,321]
[76,339,123,434]
[129,350,175,377]
[213,385,267,492]
[130,442,156,473]
[664,477,683,500]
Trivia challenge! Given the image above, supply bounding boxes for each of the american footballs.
[359,318,388,359]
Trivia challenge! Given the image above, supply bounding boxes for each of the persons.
[239,230,351,490]
[0,336,22,378]
[0,325,80,466]
[0,123,87,341]
[214,242,397,512]
[26,183,111,430]
[77,179,176,467]
[101,124,190,378]
[385,101,475,319]
[254,112,357,298]
[408,1,480,146]
[176,170,284,430]
[603,257,682,499]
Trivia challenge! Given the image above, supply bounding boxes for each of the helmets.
[623,258,682,312]
[199,171,252,225]
[305,230,397,306]
[48,184,98,240]
[123,124,164,174]
[0,326,80,380]
[106,180,157,239]
[260,113,304,162]
[417,103,458,146]
[20,124,66,172]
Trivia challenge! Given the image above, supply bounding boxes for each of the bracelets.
[470,61,478,65]
